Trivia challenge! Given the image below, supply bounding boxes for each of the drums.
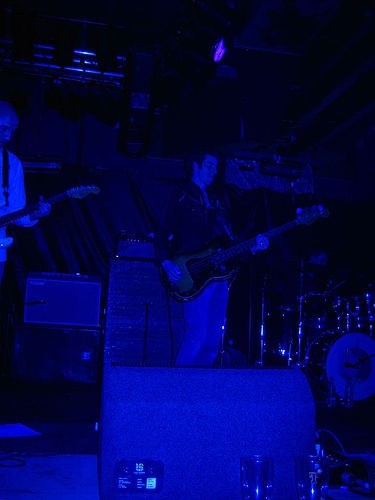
[310,330,375,402]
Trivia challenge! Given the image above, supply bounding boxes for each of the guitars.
[0,185,100,247]
[161,202,328,303]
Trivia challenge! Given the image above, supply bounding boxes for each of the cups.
[296,457,328,500]
[240,455,272,500]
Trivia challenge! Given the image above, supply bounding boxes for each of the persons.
[154,140,269,367]
[0,101,52,286]
